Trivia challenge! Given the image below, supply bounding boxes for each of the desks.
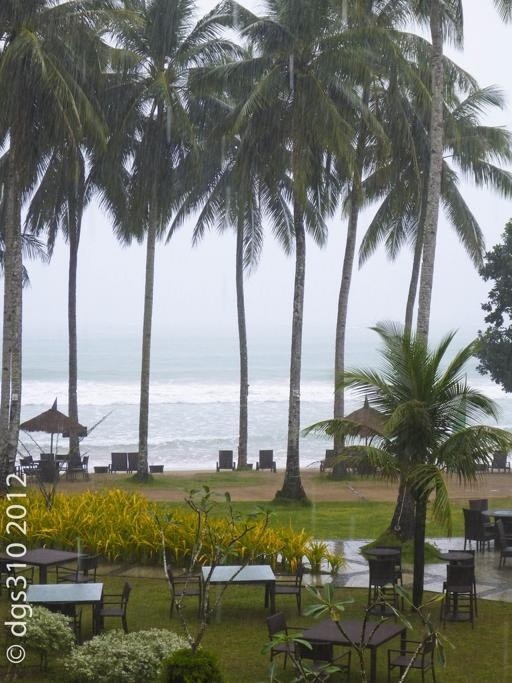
[149,465,164,473]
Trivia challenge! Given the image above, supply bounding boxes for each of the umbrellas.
[18,398,88,462]
[332,396,390,446]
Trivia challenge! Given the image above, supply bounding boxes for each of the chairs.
[319,449,334,472]
[256,449,276,472]
[215,450,236,471]
[14,449,138,481]
[0,497,511,682]
[487,450,511,473]
